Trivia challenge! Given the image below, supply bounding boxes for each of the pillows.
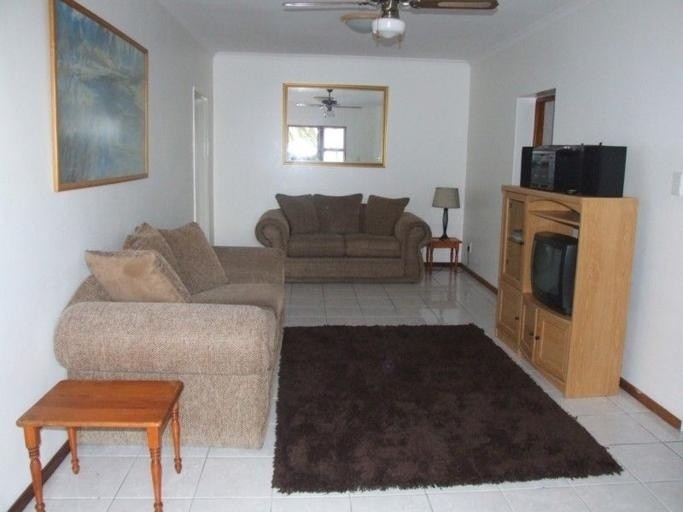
[314,193,363,233]
[84,249,192,303]
[362,195,410,236]
[123,222,193,295]
[275,194,321,235]
[157,221,230,294]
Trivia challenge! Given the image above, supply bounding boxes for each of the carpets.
[271,324,623,495]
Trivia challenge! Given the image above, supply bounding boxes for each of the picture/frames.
[47,0,149,192]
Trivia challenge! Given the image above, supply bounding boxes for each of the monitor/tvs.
[528,229,579,315]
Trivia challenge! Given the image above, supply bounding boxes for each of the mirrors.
[281,83,389,169]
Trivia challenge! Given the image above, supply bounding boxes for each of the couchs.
[53,248,286,450]
[255,203,432,284]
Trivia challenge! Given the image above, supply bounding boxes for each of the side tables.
[16,380,184,512]
[425,237,462,276]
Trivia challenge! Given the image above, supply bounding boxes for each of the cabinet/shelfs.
[497,184,639,399]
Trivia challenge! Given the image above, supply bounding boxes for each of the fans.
[282,0,500,39]
[296,89,363,118]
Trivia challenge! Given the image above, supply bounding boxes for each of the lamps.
[432,187,460,240]
[372,18,407,39]
